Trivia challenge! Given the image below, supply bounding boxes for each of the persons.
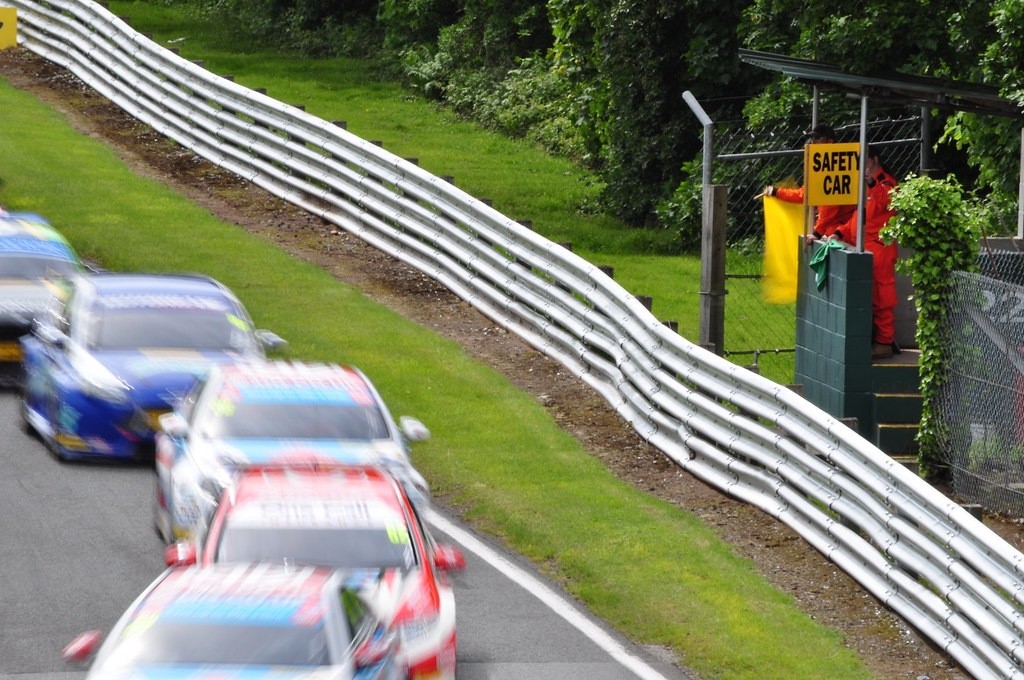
[759,123,900,361]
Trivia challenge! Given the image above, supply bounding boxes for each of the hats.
[805,125,835,139]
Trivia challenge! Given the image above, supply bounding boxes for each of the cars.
[18,273,286,464]
[0,213,87,387]
[153,364,432,547]
[62,465,465,680]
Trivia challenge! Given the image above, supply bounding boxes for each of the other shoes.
[871,340,893,359]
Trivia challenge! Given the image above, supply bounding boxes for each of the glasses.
[811,135,827,142]
